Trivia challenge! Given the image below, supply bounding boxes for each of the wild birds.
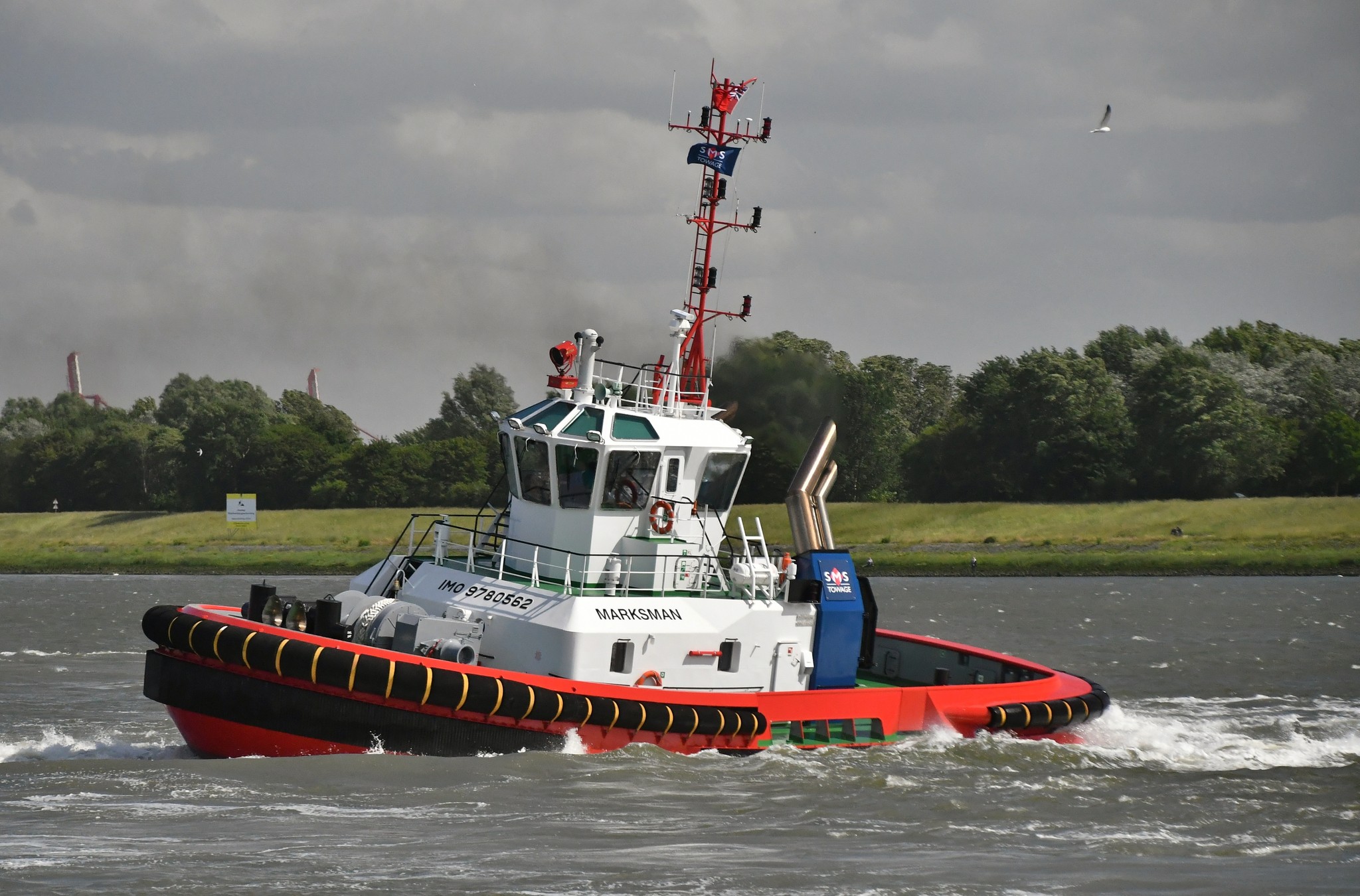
[112,571,119,576]
[197,448,203,456]
[1089,103,1112,134]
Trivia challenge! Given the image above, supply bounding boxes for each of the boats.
[139,52,1113,762]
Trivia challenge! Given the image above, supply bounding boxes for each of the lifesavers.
[637,670,662,686]
[649,500,674,534]
[615,478,638,507]
[779,552,791,584]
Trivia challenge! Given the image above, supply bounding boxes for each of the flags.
[687,143,742,177]
[729,84,749,102]
